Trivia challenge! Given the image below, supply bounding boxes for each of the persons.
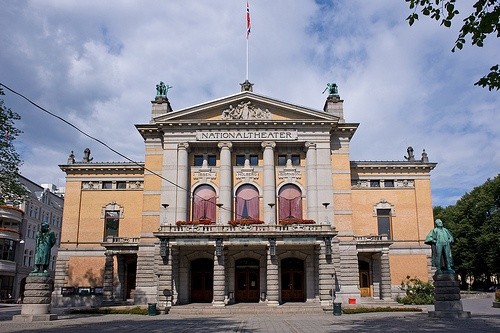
[30,221,56,273]
[424,219,456,274]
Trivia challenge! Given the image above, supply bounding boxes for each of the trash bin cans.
[148,302,157,315]
[333,302,342,316]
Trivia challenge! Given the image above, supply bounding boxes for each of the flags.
[247,0,251,39]
